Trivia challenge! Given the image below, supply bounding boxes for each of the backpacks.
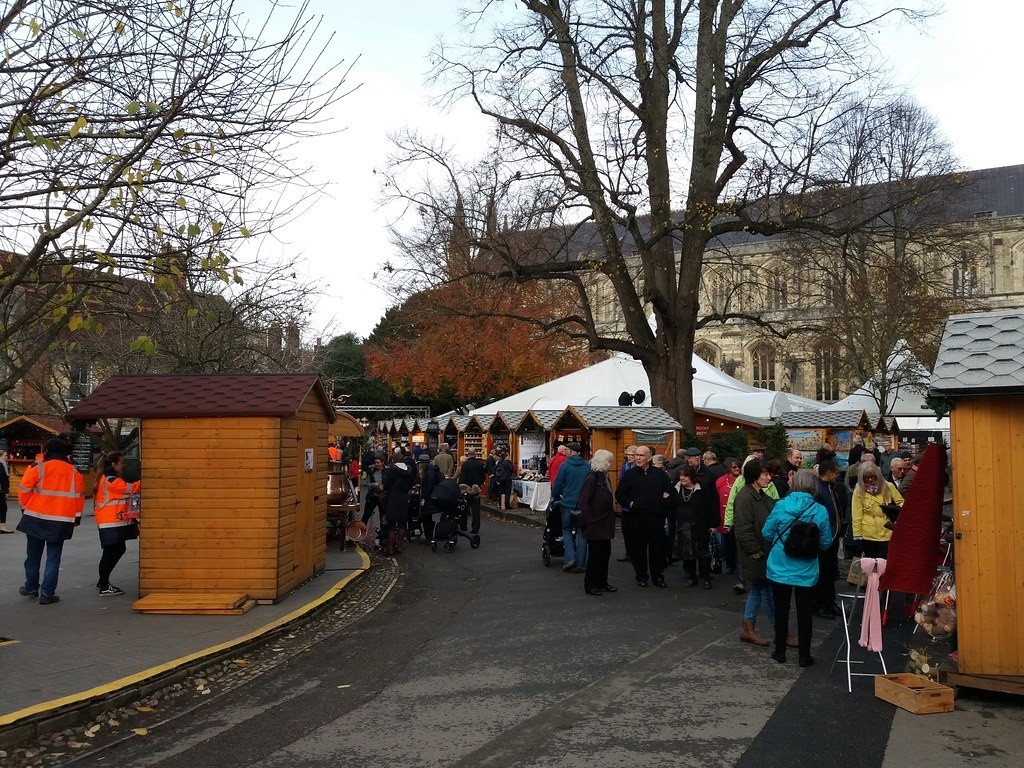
[495,461,508,482]
[783,501,820,560]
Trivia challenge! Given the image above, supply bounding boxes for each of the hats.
[566,443,581,453]
[900,451,912,459]
[685,447,701,456]
[753,447,764,451]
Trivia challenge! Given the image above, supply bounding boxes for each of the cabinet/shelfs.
[494,439,509,449]
[464,433,482,459]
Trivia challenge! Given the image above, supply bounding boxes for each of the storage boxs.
[875,673,954,715]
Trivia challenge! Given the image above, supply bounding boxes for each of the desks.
[511,480,552,511]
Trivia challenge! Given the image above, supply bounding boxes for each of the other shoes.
[0,529,14,534]
[711,568,721,575]
[705,579,712,590]
[685,577,697,588]
[563,555,668,596]
[798,656,814,668]
[470,530,478,534]
[772,651,786,663]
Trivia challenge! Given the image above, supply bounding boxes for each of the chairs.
[912,533,953,634]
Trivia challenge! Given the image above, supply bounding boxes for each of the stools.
[829,559,887,692]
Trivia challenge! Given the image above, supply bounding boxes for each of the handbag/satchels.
[710,532,726,559]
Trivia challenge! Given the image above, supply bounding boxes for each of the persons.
[540,449,550,477]
[325,439,514,555]
[547,435,923,667]
[733,457,800,650]
[760,469,834,668]
[16,439,84,605]
[0,449,14,534]
[93,451,140,597]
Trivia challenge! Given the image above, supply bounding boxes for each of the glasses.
[731,466,740,469]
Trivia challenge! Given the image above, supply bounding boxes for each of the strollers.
[431,478,481,552]
[542,499,577,566]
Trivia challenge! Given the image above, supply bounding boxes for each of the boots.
[740,619,768,646]
[773,624,799,647]
[396,528,403,555]
[381,531,394,557]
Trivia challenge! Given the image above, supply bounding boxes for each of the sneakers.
[39,594,60,604]
[19,586,39,598]
[96,580,126,597]
